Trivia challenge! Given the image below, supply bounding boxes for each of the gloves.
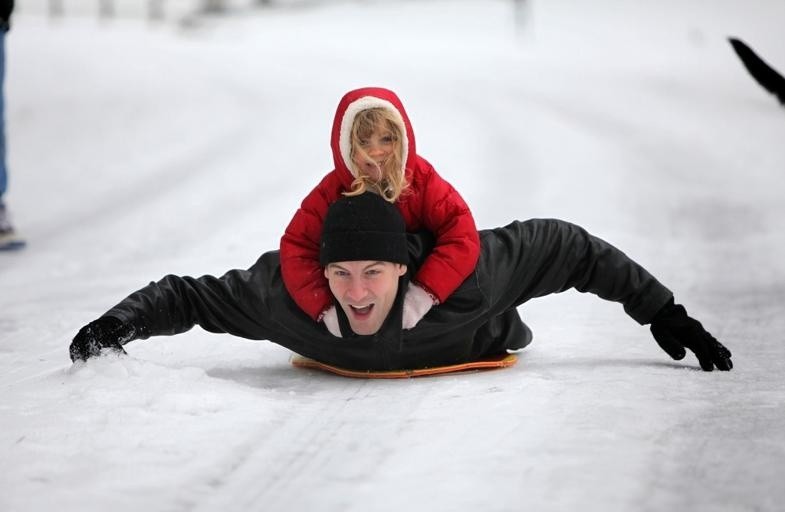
[322,307,343,340]
[650,303,735,373]
[400,282,434,332]
[69,316,133,364]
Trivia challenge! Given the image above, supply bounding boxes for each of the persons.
[277,87,481,341]
[68,217,734,373]
[1,0,30,252]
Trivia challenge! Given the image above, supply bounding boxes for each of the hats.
[321,191,410,269]
